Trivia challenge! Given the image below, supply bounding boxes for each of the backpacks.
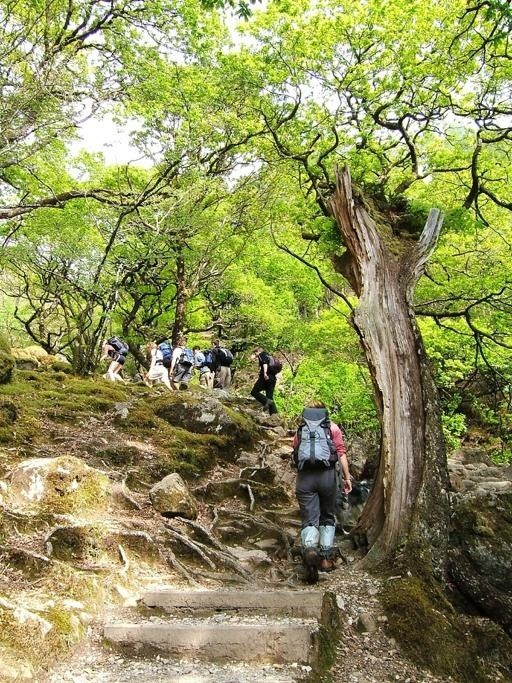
[199,349,218,370]
[155,342,172,368]
[213,347,234,364]
[289,406,339,473]
[176,347,194,367]
[105,335,130,357]
[258,351,282,374]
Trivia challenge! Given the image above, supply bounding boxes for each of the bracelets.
[344,477,352,480]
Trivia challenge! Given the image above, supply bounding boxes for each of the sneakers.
[299,547,320,585]
[262,397,274,411]
[320,558,337,573]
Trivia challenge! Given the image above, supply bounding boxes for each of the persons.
[144,341,172,392]
[100,339,126,383]
[192,347,211,389]
[163,337,172,354]
[156,340,173,369]
[212,339,231,388]
[293,400,352,586]
[251,347,277,418]
[169,338,192,391]
